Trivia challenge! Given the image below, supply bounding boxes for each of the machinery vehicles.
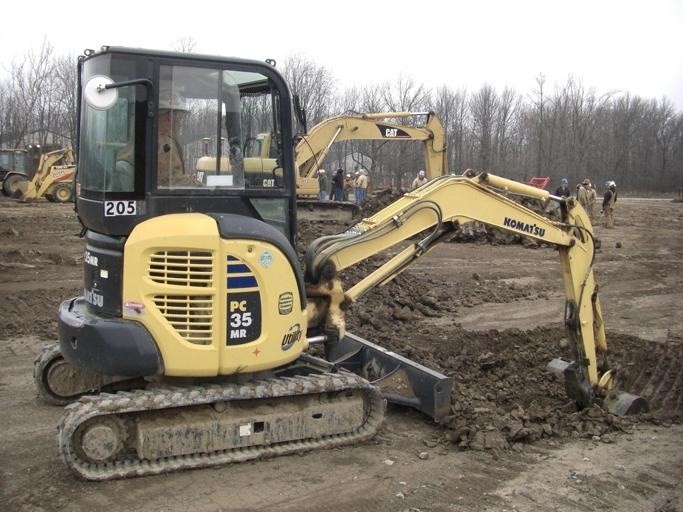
[196,109,449,227]
[0,141,77,203]
[31,44,654,486]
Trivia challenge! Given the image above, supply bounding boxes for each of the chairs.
[114,160,135,192]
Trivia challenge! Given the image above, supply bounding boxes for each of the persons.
[316,166,368,207]
[411,169,428,190]
[554,177,617,228]
[115,85,206,188]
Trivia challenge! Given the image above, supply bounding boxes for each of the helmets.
[317,169,326,173]
[346,173,351,178]
[419,171,424,175]
[355,169,363,175]
[333,168,343,176]
[576,179,591,190]
[605,181,616,189]
[560,179,568,184]
